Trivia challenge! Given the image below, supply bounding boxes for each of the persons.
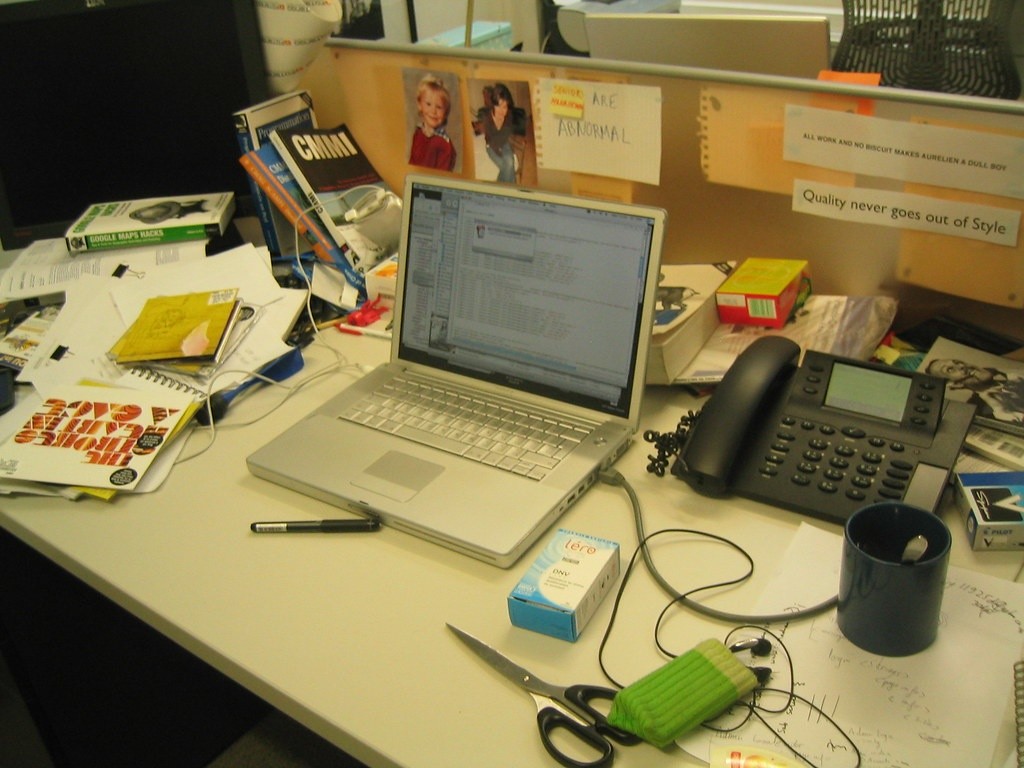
[476,84,527,184]
[407,75,458,172]
[926,358,1024,426]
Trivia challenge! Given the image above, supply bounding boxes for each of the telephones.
[670,332,979,523]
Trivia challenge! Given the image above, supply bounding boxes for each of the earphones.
[728,638,772,656]
[747,667,772,683]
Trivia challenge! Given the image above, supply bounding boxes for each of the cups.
[836,502,953,657]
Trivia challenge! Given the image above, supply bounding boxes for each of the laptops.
[583,13,830,80]
[245,174,667,568]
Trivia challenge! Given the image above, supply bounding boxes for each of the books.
[644,258,846,386]
[0,91,397,504]
[916,336,1024,484]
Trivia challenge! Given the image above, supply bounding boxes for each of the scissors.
[443,621,647,768]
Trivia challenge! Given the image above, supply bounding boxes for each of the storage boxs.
[507,531,619,642]
[717,259,813,329]
[420,20,513,52]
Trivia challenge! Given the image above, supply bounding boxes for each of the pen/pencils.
[248,515,383,534]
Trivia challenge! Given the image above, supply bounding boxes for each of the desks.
[0,0,1023,768]
